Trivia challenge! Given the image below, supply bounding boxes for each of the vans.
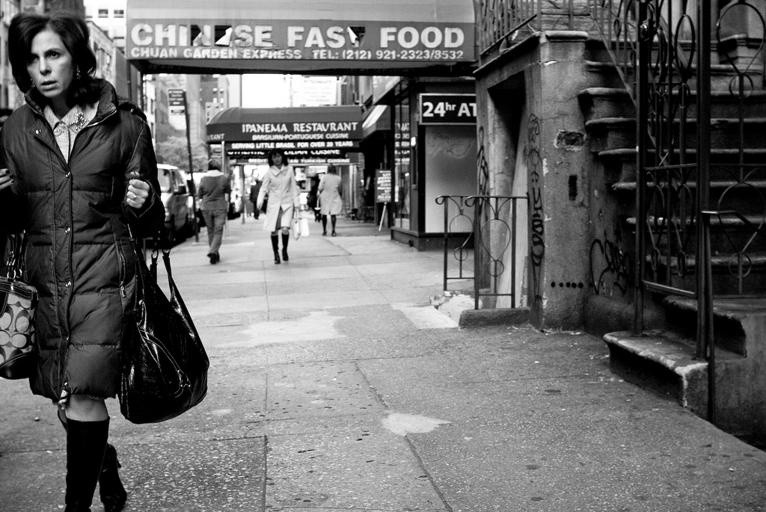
[157,164,188,249]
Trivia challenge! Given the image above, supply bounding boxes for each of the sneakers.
[322,230,337,237]
[207,251,220,264]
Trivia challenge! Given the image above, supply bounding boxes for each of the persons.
[198,149,344,264]
[0,13,164,512]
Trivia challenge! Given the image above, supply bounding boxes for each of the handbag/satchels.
[115,178,208,426]
[0,221,40,380]
[293,208,310,240]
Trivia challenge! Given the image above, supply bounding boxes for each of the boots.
[56,409,129,512]
[281,233,289,261]
[271,233,280,264]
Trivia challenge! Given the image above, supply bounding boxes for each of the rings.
[133,195,136,201]
